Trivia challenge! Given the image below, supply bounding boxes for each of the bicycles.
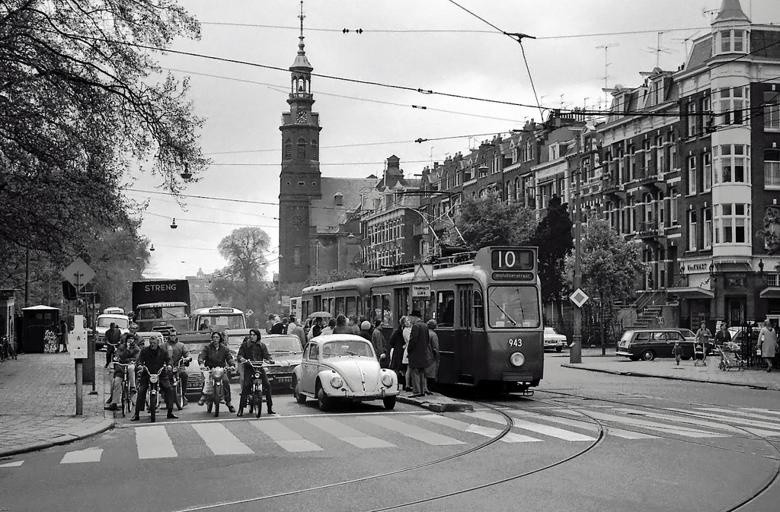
[0,335,17,362]
[112,361,139,417]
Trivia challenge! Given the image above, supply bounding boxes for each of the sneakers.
[198,397,204,405]
[227,402,236,412]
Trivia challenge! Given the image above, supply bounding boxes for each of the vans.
[614,330,713,361]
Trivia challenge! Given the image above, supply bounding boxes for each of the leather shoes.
[104,397,117,410]
[407,393,425,397]
[237,406,242,416]
[130,414,139,421]
[167,412,178,418]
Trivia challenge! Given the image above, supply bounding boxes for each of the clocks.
[295,109,308,124]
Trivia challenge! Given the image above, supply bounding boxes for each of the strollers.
[694,339,710,367]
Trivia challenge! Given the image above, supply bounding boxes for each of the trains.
[301,245,544,400]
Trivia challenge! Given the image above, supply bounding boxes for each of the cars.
[94,279,304,400]
[645,327,779,355]
[289,334,399,412]
[542,326,567,353]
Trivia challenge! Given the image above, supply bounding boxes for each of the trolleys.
[713,340,745,371]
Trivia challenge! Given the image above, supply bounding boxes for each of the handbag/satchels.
[202,371,215,395]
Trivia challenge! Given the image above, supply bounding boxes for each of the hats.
[361,321,371,329]
[409,311,422,317]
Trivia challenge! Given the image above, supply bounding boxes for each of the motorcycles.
[137,363,171,422]
[160,358,190,409]
[239,358,275,418]
[200,364,235,416]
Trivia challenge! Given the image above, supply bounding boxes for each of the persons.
[757,319,777,371]
[60,320,69,352]
[237,328,276,417]
[694,320,713,358]
[53,324,60,344]
[198,330,236,413]
[695,345,703,360]
[714,322,732,368]
[266,308,440,397]
[2,335,17,360]
[199,319,211,331]
[105,322,192,421]
[672,342,684,365]
